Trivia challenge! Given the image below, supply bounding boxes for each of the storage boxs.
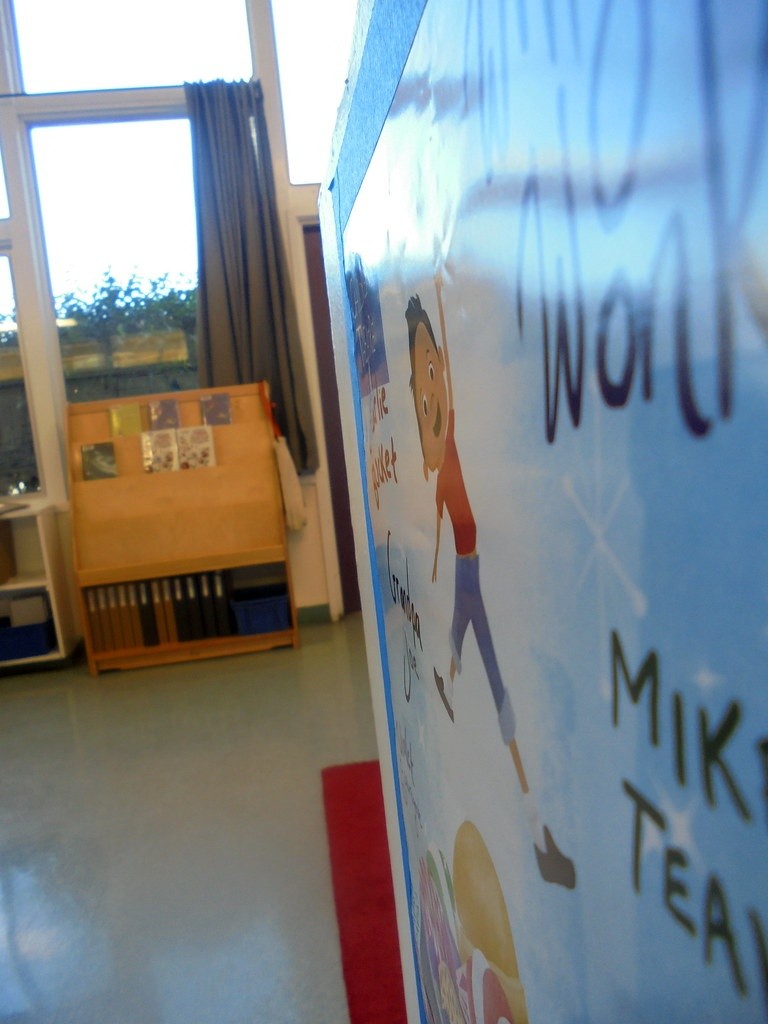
[0,617,53,662]
[231,583,291,635]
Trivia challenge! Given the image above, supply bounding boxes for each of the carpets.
[321,759,408,1024]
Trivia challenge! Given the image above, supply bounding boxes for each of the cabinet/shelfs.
[0,505,77,668]
